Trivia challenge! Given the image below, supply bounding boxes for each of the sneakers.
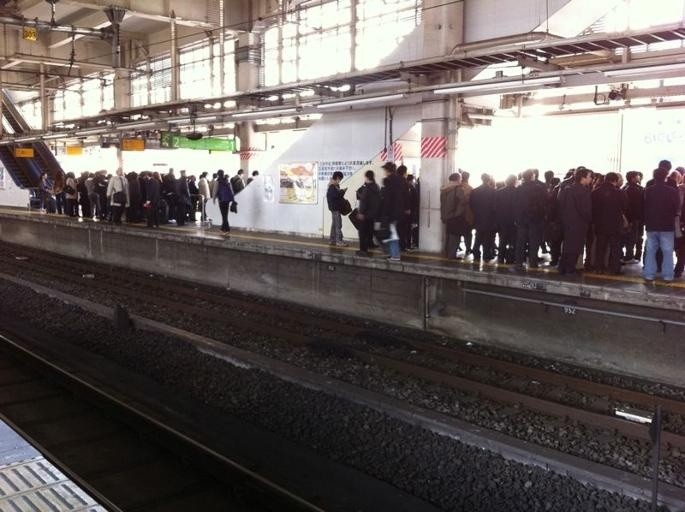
[385,256,402,263]
[329,240,349,248]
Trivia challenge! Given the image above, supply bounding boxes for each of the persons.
[440,159,684,286]
[325,162,421,262]
[37,167,260,236]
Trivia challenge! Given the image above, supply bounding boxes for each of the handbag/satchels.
[230,200,239,214]
[339,197,352,216]
[112,190,127,205]
[53,185,76,195]
[348,208,361,230]
